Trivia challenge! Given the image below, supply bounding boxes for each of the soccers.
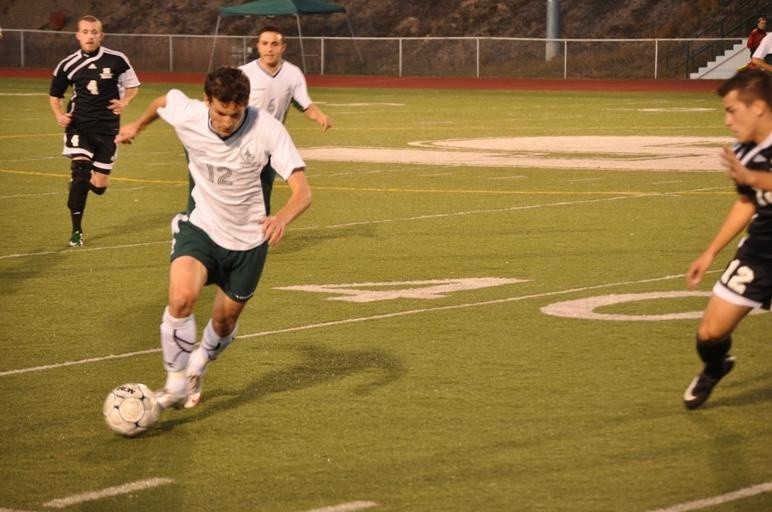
[103,384,159,436]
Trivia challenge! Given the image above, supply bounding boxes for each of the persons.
[47,16,141,246]
[239,27,336,134]
[114,66,313,410]
[752,32,772,74]
[747,17,768,58]
[685,68,772,409]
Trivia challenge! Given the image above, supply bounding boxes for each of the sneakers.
[183,351,204,410]
[151,386,187,410]
[682,352,736,411]
[69,229,83,248]
